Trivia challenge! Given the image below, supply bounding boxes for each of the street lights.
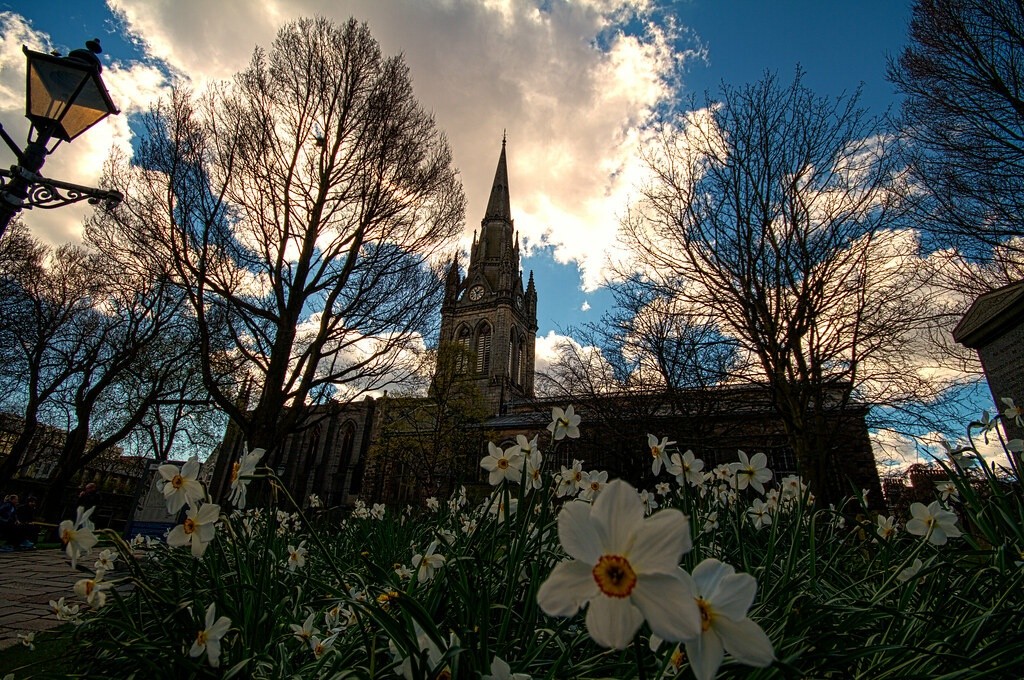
[0,38,124,240]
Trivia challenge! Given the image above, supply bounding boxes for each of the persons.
[72,481,101,529]
[0,494,41,551]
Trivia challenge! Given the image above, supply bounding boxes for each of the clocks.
[516,293,524,312]
[468,284,486,301]
